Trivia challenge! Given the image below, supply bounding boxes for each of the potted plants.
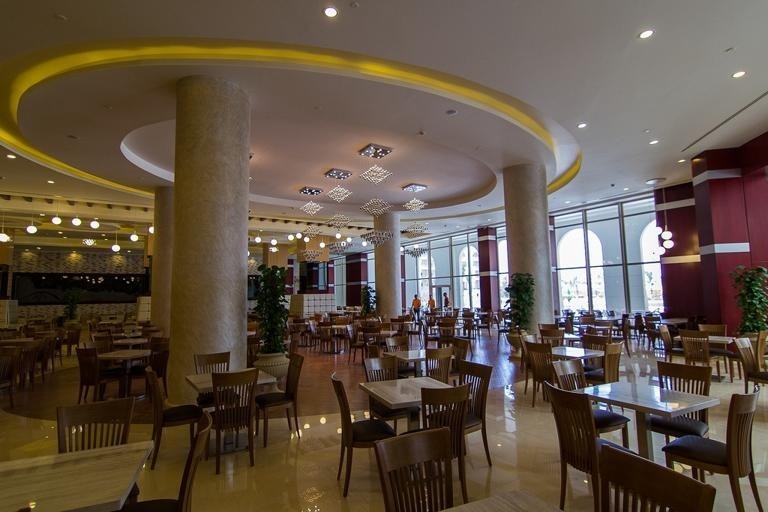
[502,273,534,361]
[731,262,767,356]
[253,262,290,381]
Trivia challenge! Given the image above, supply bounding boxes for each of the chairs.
[256,352,305,447]
[205,368,259,475]
[550,358,630,448]
[75,347,118,403]
[330,371,396,497]
[127,343,170,399]
[540,382,638,512]
[1,295,768,378]
[648,360,712,481]
[733,337,768,392]
[1,346,23,407]
[364,357,421,433]
[193,351,242,411]
[662,390,763,512]
[427,359,494,480]
[599,444,715,512]
[137,408,213,512]
[145,366,203,471]
[525,341,555,413]
[55,396,136,454]
[114,447,154,512]
[373,426,455,512]
[421,382,473,503]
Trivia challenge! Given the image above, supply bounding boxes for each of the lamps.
[325,167,354,257]
[300,187,323,265]
[404,183,429,259]
[644,178,673,258]
[0,193,279,253]
[358,142,395,249]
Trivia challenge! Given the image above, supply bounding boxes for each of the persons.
[428,295,435,312]
[444,293,449,307]
[411,294,421,321]
